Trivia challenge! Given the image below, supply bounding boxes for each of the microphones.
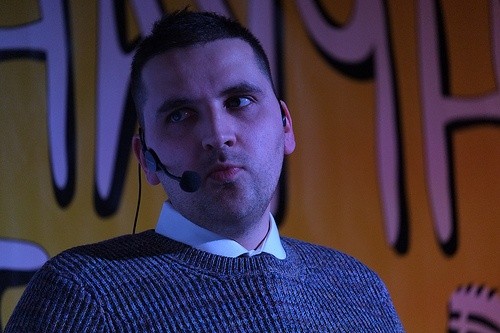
[144,149,202,192]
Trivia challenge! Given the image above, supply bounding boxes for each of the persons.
[4,4,408,333]
